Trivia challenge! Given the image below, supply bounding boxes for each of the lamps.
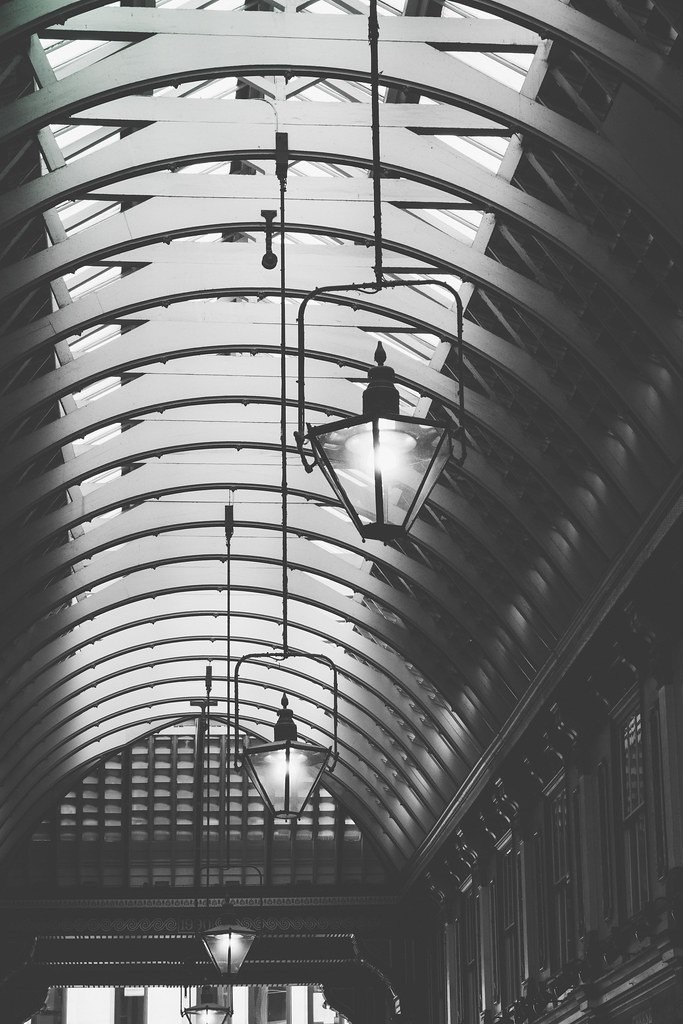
[180,665,233,1024]
[294,0,468,537]
[194,504,271,976]
[232,130,342,820]
